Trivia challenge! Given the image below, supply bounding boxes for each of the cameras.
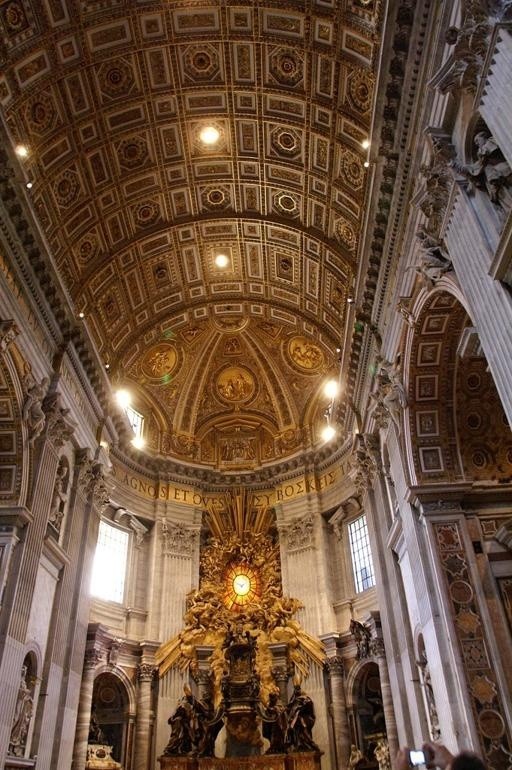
[408,750,433,766]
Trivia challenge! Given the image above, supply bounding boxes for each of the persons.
[8,665,32,757]
[416,646,438,726]
[355,0,512,489]
[163,534,315,758]
[374,738,392,770]
[89,704,102,742]
[137,663,159,678]
[150,343,320,398]
[349,618,371,659]
[396,741,487,770]
[109,636,118,666]
[224,440,256,460]
[347,744,362,770]
[23,377,110,523]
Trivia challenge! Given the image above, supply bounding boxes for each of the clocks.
[232,574,251,596]
